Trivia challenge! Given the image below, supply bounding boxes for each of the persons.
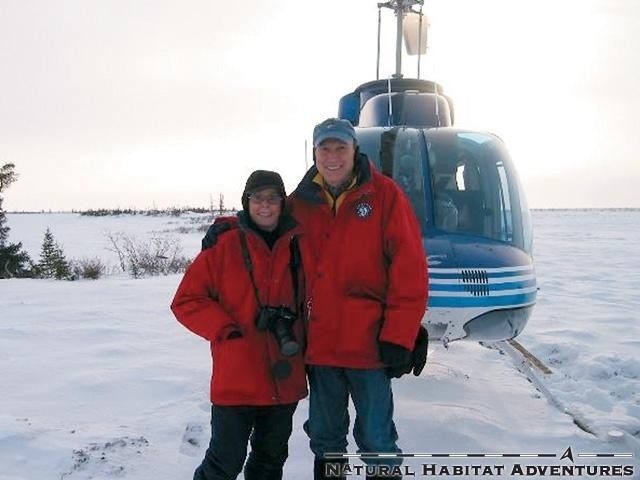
[200,117,429,480]
[170,169,309,480]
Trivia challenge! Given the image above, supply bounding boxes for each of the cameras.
[256,304,300,357]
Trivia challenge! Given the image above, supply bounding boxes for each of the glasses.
[248,194,282,205]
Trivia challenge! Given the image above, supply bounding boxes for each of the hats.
[313,118,357,149]
[242,170,286,210]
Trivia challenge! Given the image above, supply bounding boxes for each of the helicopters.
[339,1,555,380]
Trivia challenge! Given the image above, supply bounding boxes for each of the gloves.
[382,341,411,374]
[409,325,428,376]
[202,223,231,250]
